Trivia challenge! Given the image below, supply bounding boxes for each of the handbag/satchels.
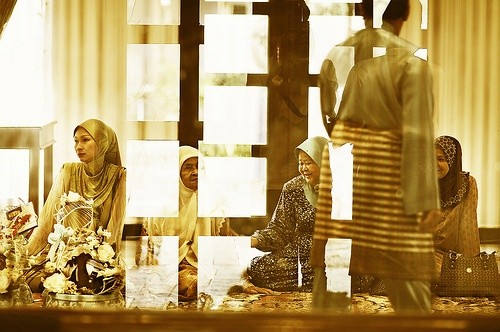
[436,250,500,297]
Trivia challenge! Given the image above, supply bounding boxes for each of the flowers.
[42,191,121,294]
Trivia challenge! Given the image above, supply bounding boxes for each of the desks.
[0,120,58,218]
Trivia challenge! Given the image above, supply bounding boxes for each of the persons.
[432,136,480,259]
[311,0,442,312]
[136,146,273,302]
[25,119,126,292]
[234,137,383,292]
